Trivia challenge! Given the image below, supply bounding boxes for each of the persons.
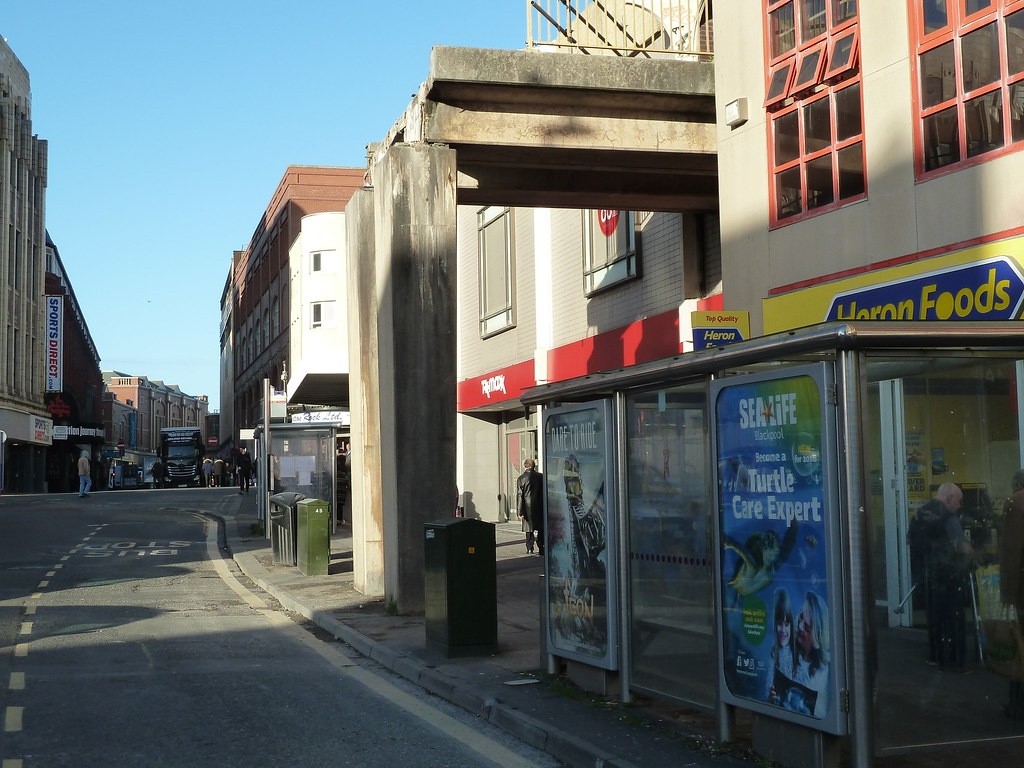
[151,458,163,489]
[77,450,92,498]
[765,588,829,718]
[337,455,350,526]
[908,470,1024,675]
[516,459,545,555]
[237,445,251,495]
[202,456,232,488]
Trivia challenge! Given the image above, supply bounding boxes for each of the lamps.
[725,97,747,126]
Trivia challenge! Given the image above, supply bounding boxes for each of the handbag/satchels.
[984,600,1024,682]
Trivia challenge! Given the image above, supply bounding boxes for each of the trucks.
[156,427,205,486]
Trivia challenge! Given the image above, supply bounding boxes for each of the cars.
[547,450,696,535]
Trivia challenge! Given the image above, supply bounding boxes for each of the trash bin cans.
[269,490,307,567]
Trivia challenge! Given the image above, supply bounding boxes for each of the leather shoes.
[539,550,544,554]
[525,550,533,554]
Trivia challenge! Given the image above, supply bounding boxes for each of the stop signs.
[117,442,124,449]
[208,437,218,446]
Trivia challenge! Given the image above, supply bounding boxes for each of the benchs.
[630,616,714,674]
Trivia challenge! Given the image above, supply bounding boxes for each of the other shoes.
[924,656,941,666]
[208,485,211,488]
[239,491,244,496]
[219,485,221,487]
[82,491,89,497]
[947,663,976,675]
[214,485,218,487]
[337,519,346,525]
[245,493,248,495]
